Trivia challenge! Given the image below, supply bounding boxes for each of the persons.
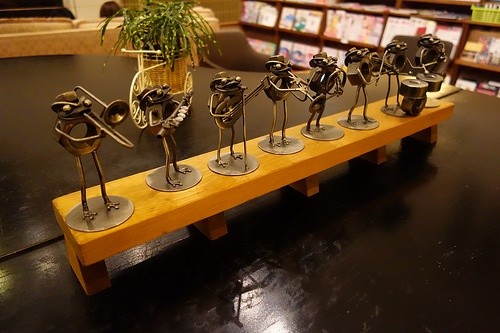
[100,2,123,18]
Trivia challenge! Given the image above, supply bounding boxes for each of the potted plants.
[96,0,224,91]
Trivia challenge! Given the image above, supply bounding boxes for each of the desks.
[1,54,460,267]
[0,88,500,333]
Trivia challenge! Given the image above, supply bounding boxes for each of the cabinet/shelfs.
[240,0,500,98]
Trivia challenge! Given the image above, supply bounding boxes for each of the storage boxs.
[471,2,500,23]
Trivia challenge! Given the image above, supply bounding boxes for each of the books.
[239,0,500,98]
